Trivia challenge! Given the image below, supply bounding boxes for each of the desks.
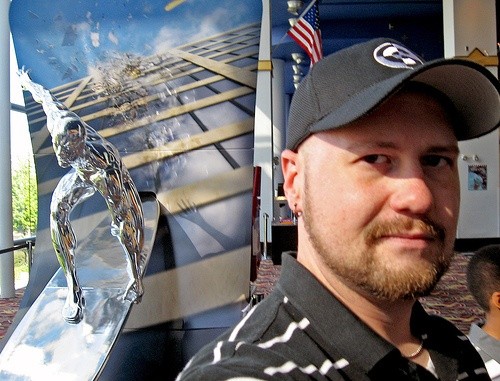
[271,226,298,265]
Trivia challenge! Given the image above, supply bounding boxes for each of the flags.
[287,0,324,66]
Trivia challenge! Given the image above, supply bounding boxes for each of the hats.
[286,38,499,151]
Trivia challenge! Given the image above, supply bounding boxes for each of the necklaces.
[400,343,424,360]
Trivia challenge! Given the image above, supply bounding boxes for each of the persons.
[172,38,500,381]
[464,244,500,381]
[14,64,146,324]
